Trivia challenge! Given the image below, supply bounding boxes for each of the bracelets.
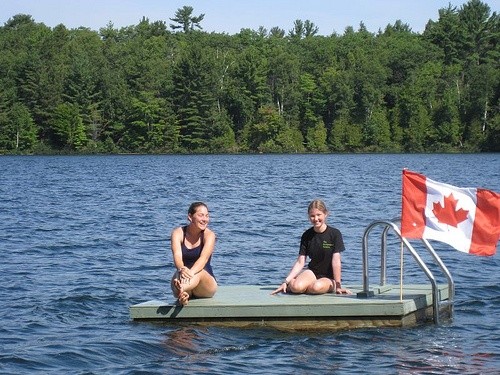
[178,268,182,273]
[282,280,289,285]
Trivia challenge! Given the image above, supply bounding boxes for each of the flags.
[401,170,500,257]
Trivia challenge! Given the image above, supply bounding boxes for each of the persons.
[171,201,217,305]
[268,199,352,295]
